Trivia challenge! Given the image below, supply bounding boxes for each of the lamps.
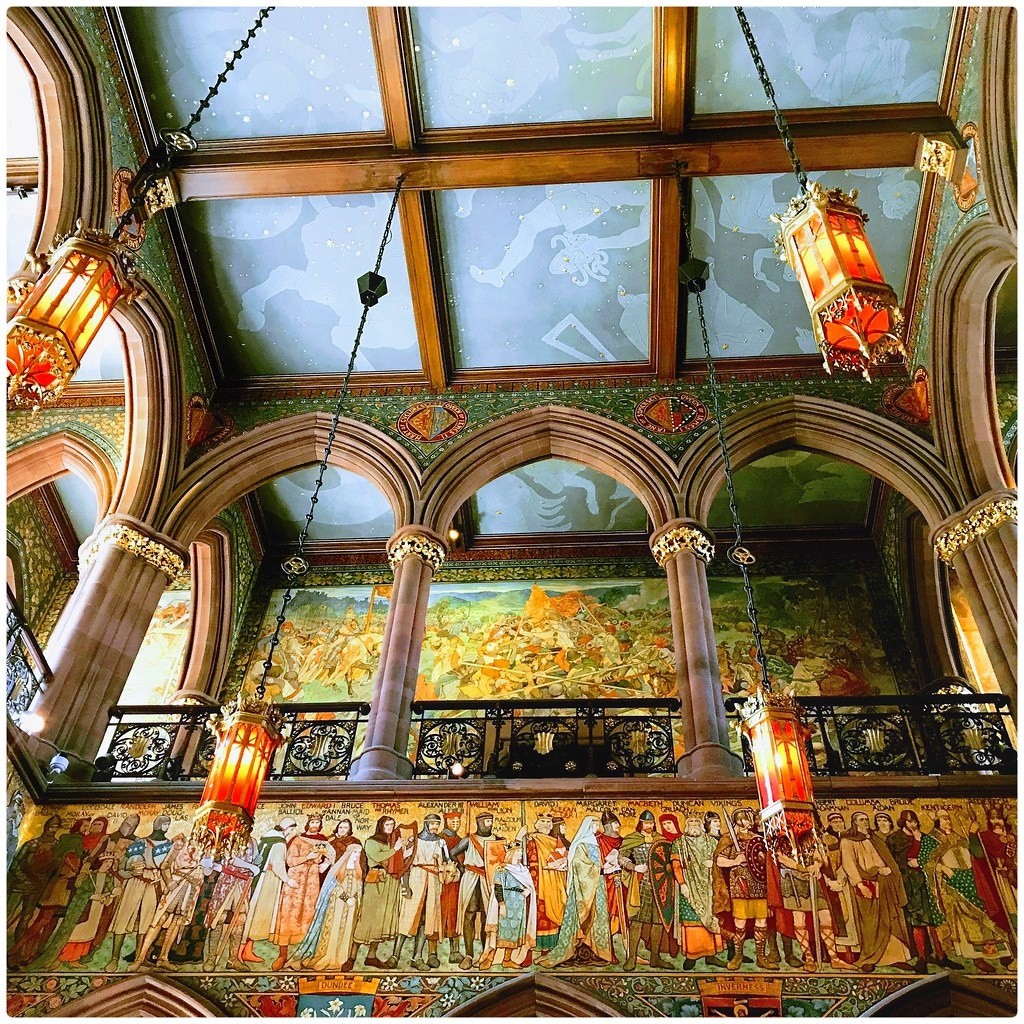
[734,6,907,382]
[6,7,277,408]
[187,176,406,855]
[676,260,820,866]
